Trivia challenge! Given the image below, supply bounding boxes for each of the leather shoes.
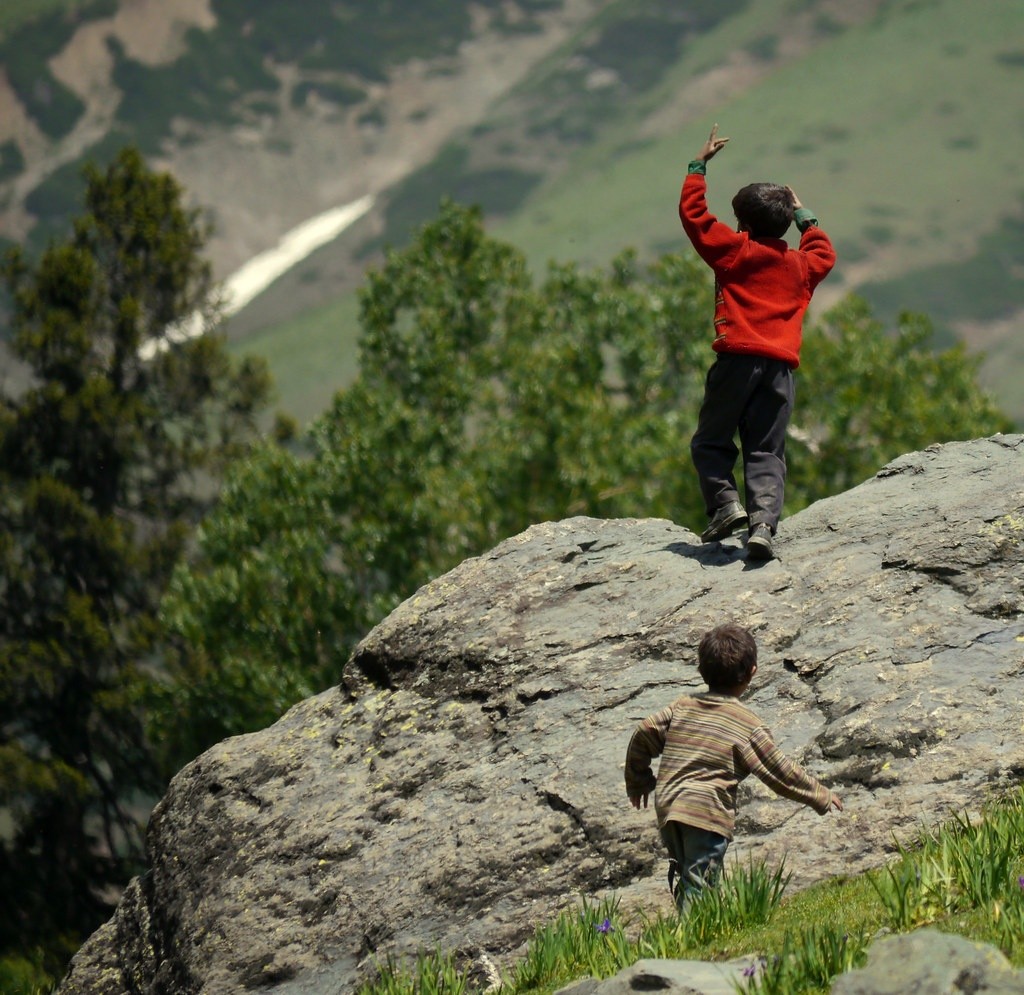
[701,500,748,543]
[748,526,774,558]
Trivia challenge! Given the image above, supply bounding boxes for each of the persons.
[677,123,836,561]
[624,622,843,920]
